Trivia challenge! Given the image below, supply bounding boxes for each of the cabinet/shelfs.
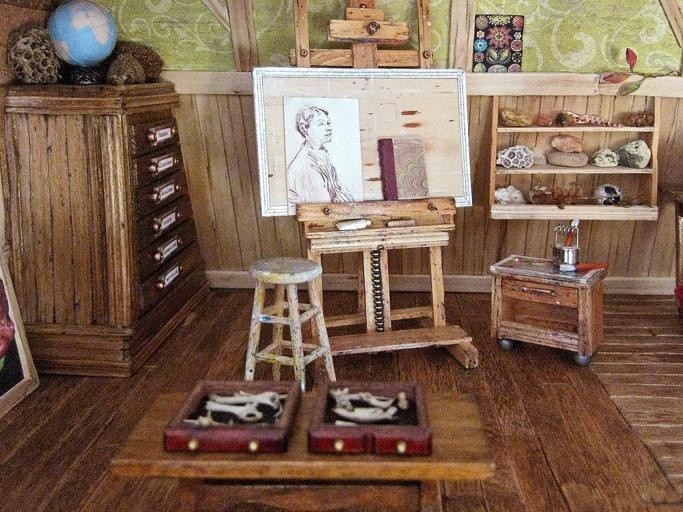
[485,248,609,369]
[2,77,215,385]
[483,94,664,222]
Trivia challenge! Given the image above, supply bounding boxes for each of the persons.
[285,105,353,216]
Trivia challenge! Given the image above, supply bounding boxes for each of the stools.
[242,255,334,393]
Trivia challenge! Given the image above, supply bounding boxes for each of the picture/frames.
[0,250,41,423]
[249,62,475,221]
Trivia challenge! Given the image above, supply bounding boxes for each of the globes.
[47,1,117,84]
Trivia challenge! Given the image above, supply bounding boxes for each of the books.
[378,136,430,200]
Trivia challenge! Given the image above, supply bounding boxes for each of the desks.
[653,179,683,325]
[106,386,498,509]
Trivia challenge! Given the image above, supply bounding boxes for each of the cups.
[550,246,580,268]
